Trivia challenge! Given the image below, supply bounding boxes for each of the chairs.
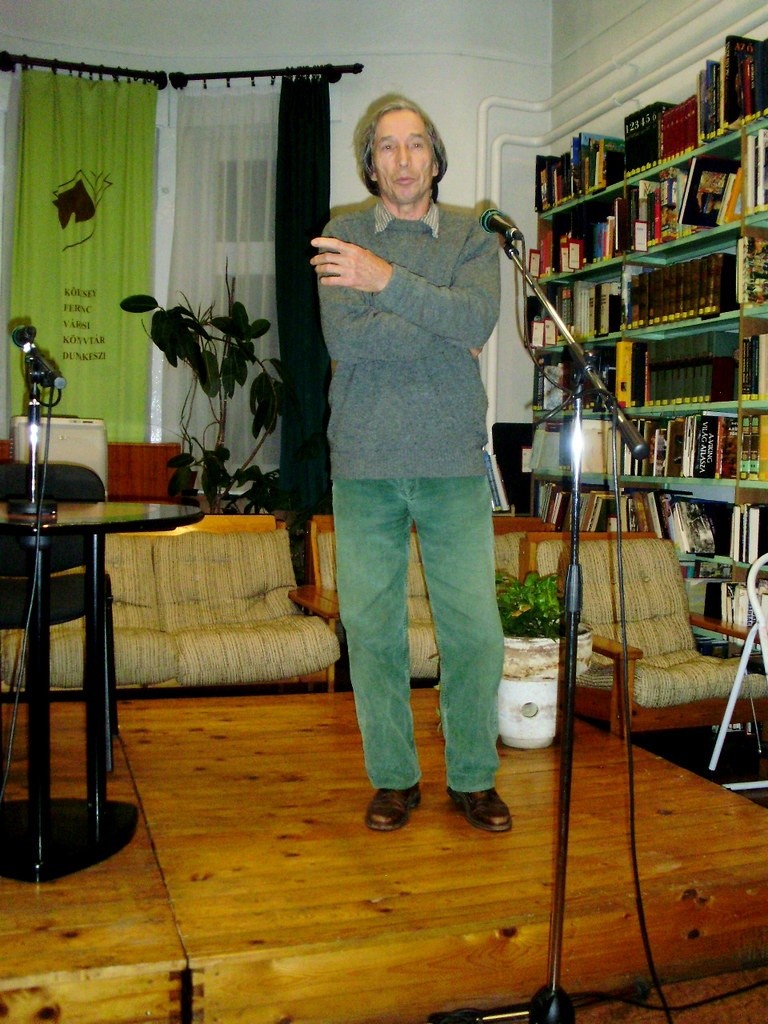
[1,523,119,775]
[515,514,768,789]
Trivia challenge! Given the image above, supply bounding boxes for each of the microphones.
[477,208,524,240]
[12,324,67,389]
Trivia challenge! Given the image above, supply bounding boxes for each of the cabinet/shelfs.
[532,117,768,650]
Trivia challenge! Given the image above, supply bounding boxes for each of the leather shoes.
[446,787,512,831]
[365,783,420,831]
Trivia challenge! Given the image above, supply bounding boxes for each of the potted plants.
[487,574,597,749]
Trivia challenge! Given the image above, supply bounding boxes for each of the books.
[528,35,768,626]
[483,450,510,511]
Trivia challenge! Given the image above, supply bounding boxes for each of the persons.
[309,99,513,832]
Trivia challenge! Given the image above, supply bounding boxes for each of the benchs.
[288,515,562,683]
[1,514,341,698]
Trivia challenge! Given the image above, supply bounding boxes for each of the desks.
[1,501,206,882]
